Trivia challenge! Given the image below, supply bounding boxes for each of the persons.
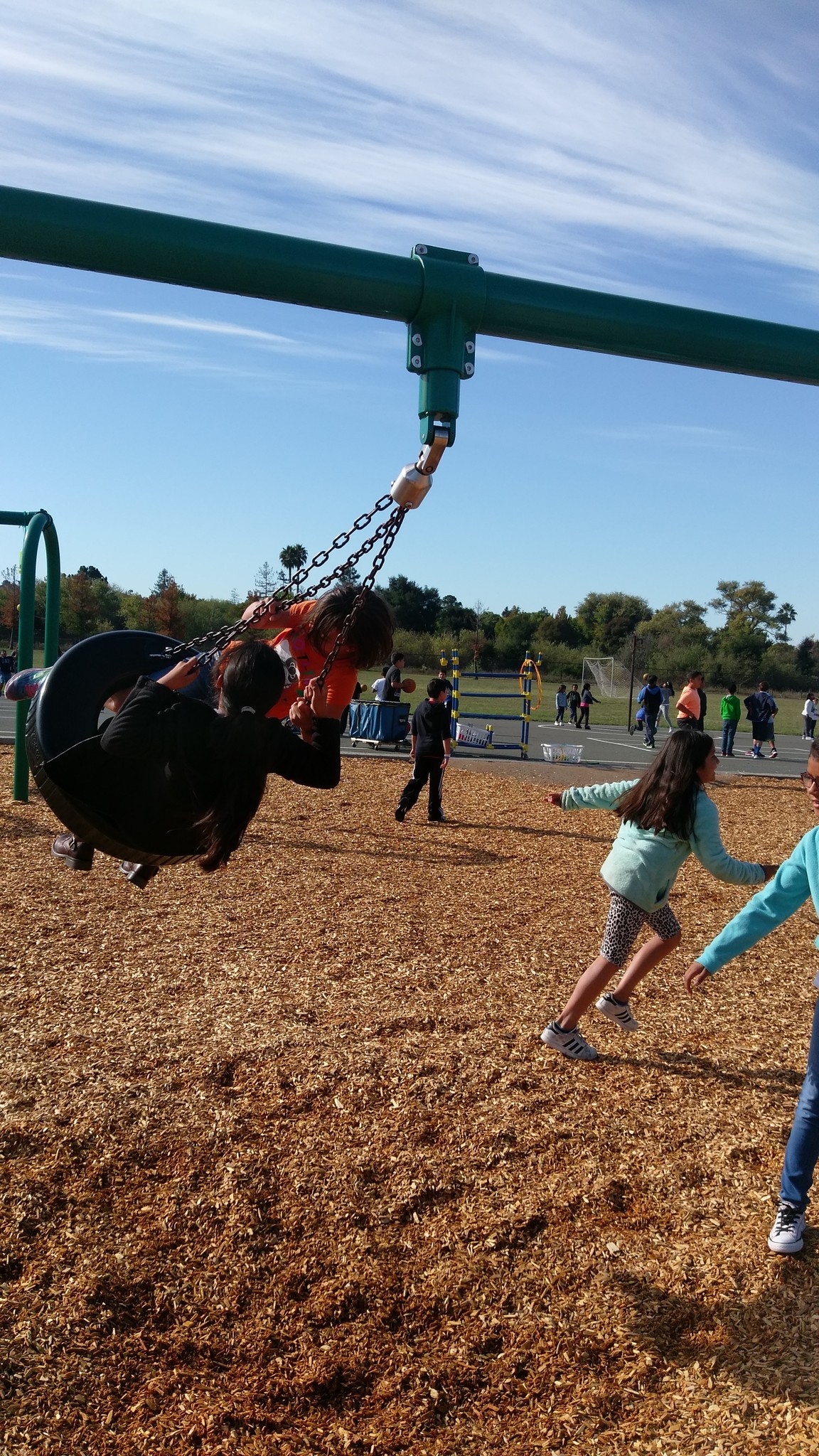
[4,584,395,742]
[554,684,568,726]
[437,670,453,725]
[0,647,19,697]
[576,683,601,730]
[743,681,778,758]
[395,678,455,822]
[630,670,707,749]
[372,653,413,748]
[52,640,341,890]
[566,684,581,726]
[540,730,780,1059]
[720,684,741,757]
[683,738,819,1253]
[801,692,819,740]
[340,682,363,737]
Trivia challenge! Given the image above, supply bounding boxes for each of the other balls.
[17,604,20,612]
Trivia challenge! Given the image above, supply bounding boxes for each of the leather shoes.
[51,832,94,872]
[118,860,160,889]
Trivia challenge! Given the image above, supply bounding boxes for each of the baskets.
[455,722,494,746]
[540,743,584,764]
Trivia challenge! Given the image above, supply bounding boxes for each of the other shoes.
[554,721,559,726]
[629,725,635,736]
[656,726,660,731]
[559,722,564,726]
[643,741,655,748]
[668,727,674,733]
[721,752,735,757]
[574,722,582,729]
[568,721,573,724]
[584,726,591,730]
[802,735,814,740]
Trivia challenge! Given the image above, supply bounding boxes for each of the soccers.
[643,674,649,681]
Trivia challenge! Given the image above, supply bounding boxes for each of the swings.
[23,436,456,866]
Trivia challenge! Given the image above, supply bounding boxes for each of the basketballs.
[401,678,416,694]
[360,684,368,692]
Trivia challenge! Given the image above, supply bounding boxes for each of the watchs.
[444,754,451,758]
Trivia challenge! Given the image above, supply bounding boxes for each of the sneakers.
[752,753,765,759]
[427,817,447,822]
[541,1021,597,1060]
[745,749,754,756]
[400,738,412,748]
[4,666,52,701]
[767,1199,807,1254]
[395,797,409,822]
[769,750,778,758]
[594,991,638,1032]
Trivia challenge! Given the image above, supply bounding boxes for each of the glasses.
[800,771,819,789]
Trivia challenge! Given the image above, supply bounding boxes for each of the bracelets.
[444,756,449,760]
